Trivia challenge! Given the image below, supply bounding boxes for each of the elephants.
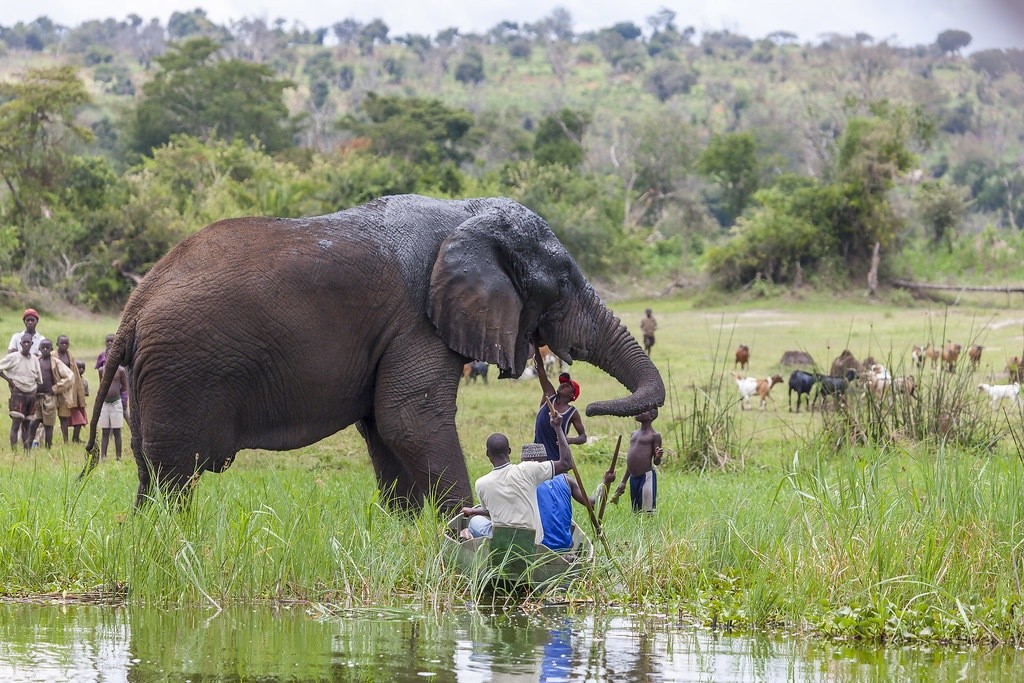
[79,194,666,544]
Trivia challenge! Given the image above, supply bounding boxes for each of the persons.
[68,360,90,444]
[640,307,658,356]
[615,408,663,517]
[8,309,46,448]
[0,334,43,455]
[50,334,80,446]
[94,334,130,462]
[461,346,615,553]
[29,339,75,450]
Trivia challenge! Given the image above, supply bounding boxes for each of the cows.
[731,340,1024,416]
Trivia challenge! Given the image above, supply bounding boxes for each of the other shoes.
[115,456,121,465]
[100,455,106,464]
[32,442,39,447]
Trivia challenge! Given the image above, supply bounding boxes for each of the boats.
[442,501,594,594]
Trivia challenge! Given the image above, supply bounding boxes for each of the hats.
[559,373,580,401]
[521,444,547,458]
[23,308,39,322]
